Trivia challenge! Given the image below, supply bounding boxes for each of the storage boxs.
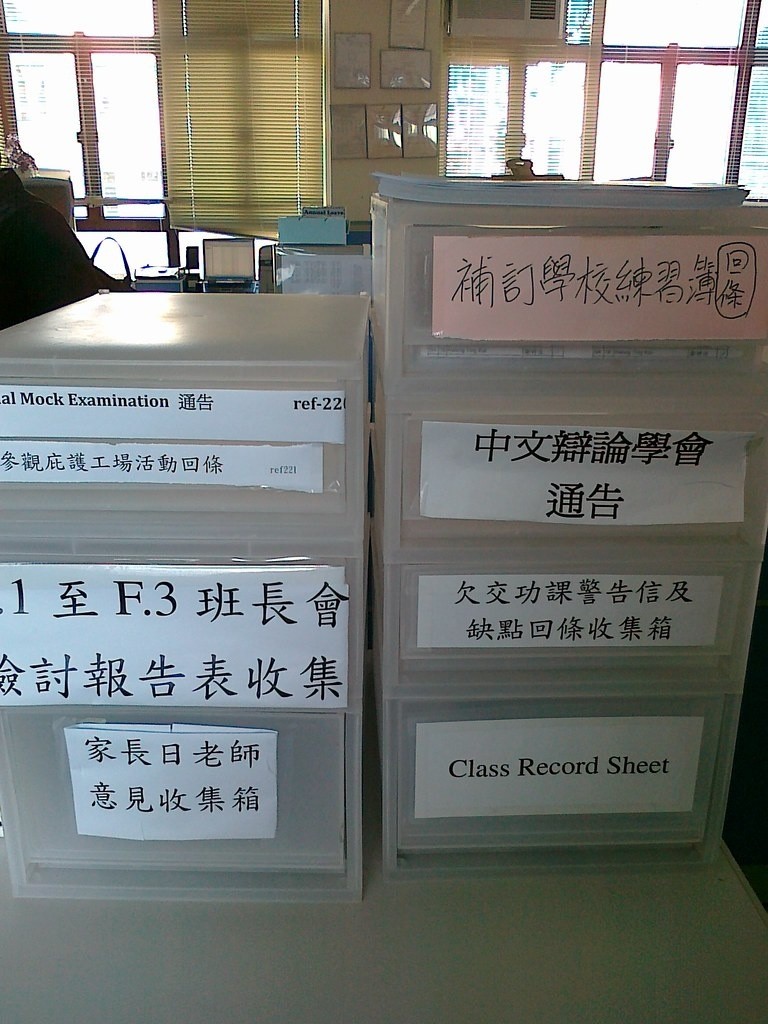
[0,206,768,905]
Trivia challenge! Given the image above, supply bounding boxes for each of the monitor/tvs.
[203,238,256,281]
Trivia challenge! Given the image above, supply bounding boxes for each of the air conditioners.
[449,3,566,39]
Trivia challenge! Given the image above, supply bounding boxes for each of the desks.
[3,841,765,1024]
